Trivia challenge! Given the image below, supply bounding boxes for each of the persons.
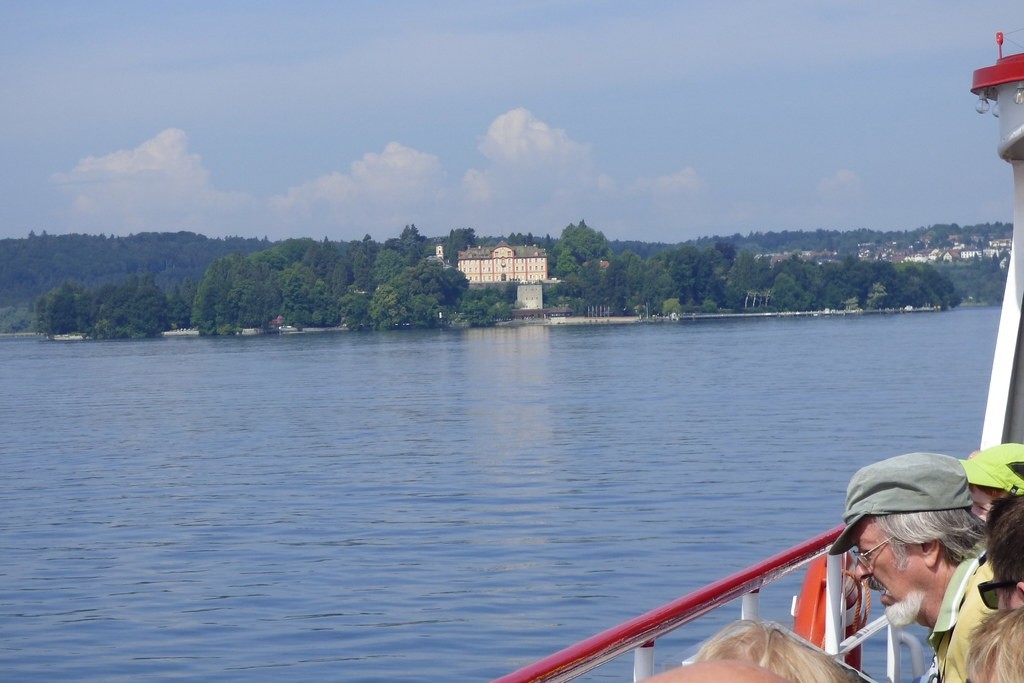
[966,608,1023,683]
[981,498,1023,612]
[828,446,1008,683]
[688,619,854,683]
[951,443,1024,522]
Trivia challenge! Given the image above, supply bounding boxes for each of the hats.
[828,451,975,555]
[957,442,1024,496]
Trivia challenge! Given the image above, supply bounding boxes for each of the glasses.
[850,536,895,567]
[978,578,1024,609]
